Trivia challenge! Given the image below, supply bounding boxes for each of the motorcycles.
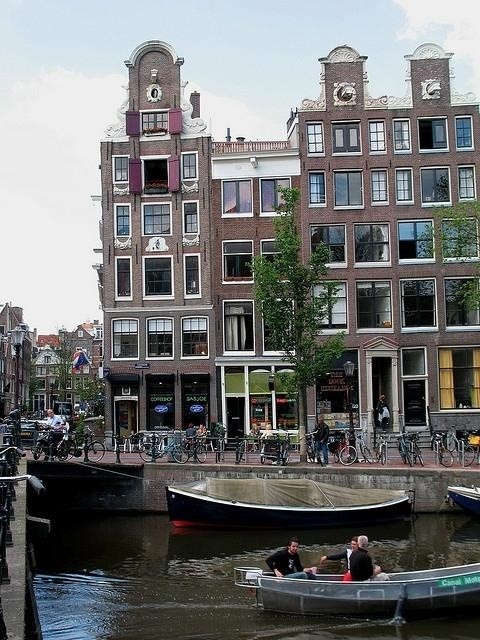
[31,421,68,461]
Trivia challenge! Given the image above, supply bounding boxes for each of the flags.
[72,352,89,368]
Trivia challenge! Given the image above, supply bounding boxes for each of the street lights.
[343,360,359,463]
[7,324,26,409]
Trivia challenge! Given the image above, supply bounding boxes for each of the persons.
[320,535,359,584]
[258,424,274,456]
[349,535,391,581]
[265,536,317,580]
[40,409,70,461]
[311,415,330,464]
[375,394,391,433]
[248,424,260,453]
[185,422,210,462]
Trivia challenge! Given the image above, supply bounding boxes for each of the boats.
[164,478,415,529]
[444,484,480,518]
[233,563,480,614]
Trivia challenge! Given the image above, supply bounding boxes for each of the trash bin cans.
[167,432,182,462]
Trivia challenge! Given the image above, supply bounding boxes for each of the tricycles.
[260,438,291,466]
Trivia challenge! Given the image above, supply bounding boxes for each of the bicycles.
[304,428,480,467]
[139,435,252,464]
[56,425,105,463]
[0,446,46,518]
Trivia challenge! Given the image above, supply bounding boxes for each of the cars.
[21,415,69,445]
